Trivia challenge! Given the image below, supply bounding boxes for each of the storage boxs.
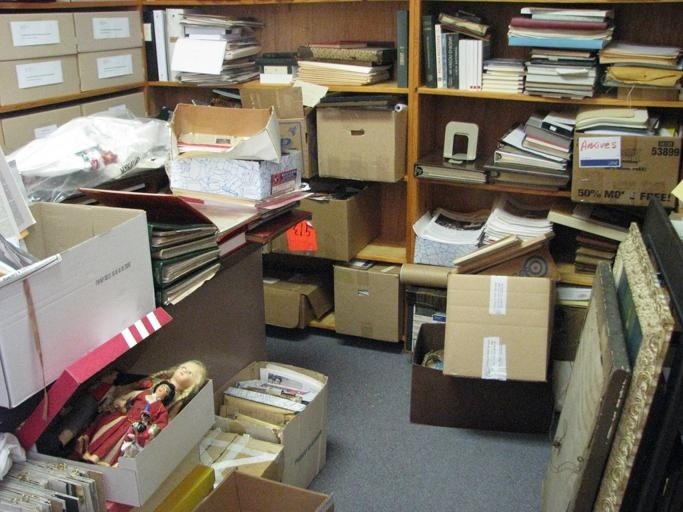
[0,201,333,511]
[408,271,561,439]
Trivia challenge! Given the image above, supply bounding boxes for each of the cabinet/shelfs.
[0,0,683,349]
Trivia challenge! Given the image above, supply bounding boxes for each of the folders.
[142,8,202,81]
[147,224,222,306]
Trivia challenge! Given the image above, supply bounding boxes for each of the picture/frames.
[539,195,683,512]
